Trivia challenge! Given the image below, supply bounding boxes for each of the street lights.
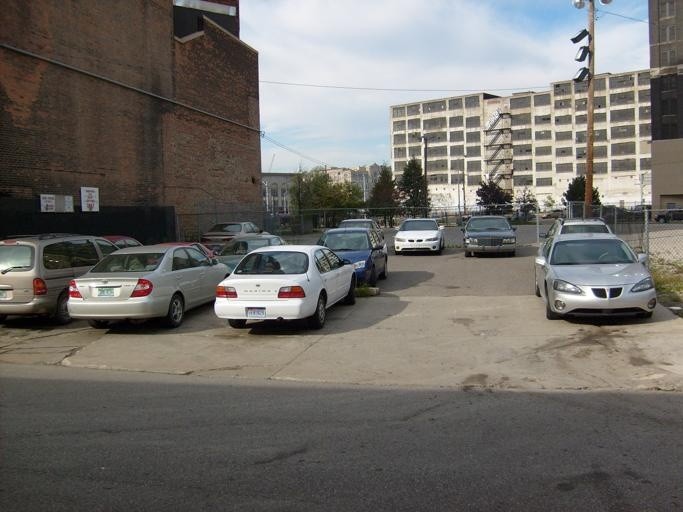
[418,135,429,218]
[570,0,612,218]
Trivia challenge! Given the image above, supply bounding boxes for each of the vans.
[0,232,120,324]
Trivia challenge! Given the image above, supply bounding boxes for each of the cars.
[461,215,517,256]
[316,218,388,286]
[627,205,683,223]
[534,218,657,319]
[104,221,287,272]
[66,245,232,329]
[393,218,445,253]
[214,246,357,329]
[460,209,564,219]
